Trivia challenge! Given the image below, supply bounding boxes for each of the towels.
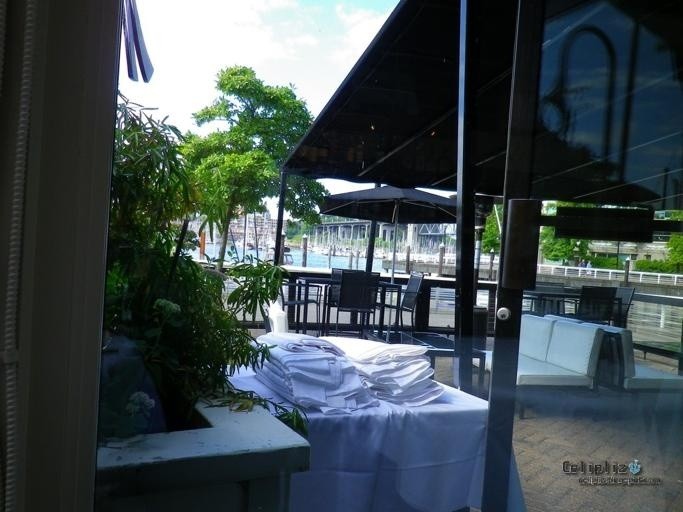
[250,332,445,415]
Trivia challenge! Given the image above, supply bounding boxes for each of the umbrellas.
[320,184,457,342]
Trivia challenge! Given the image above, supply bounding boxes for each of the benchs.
[472,314,607,419]
[544,314,683,413]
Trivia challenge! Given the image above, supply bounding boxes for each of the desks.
[227,365,527,512]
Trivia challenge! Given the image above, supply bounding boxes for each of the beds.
[362,333,486,396]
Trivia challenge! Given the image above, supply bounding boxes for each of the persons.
[577,259,593,277]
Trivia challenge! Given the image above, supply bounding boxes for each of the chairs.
[523,282,636,328]
[280,268,424,336]
